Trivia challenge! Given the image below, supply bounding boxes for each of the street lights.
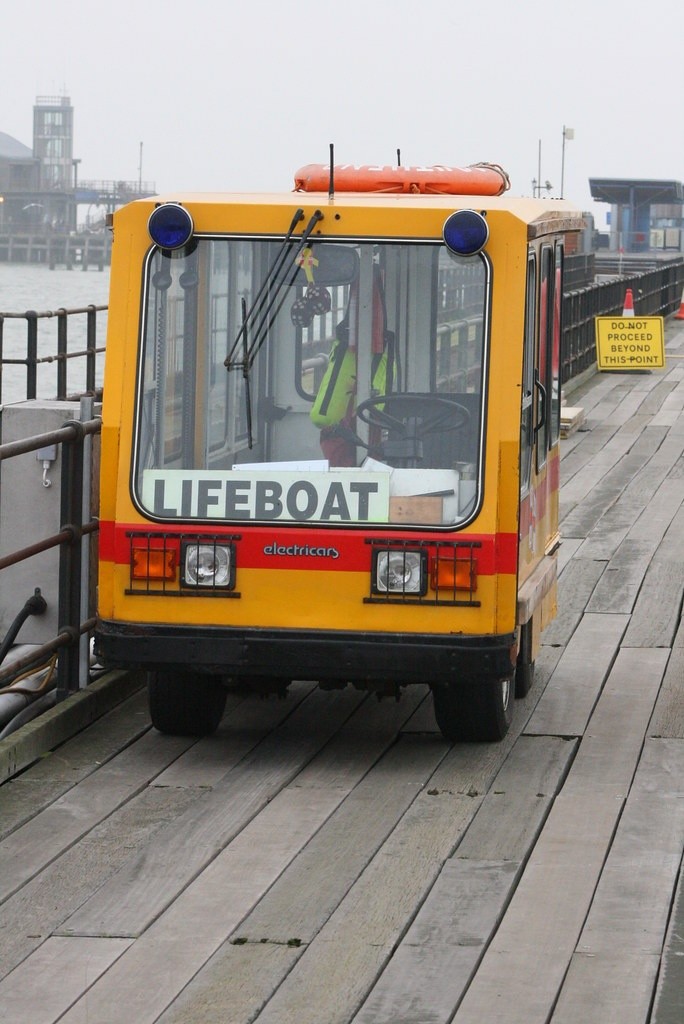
[560,124,576,198]
[531,178,537,197]
[72,158,81,233]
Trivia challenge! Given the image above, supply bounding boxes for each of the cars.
[92,143,584,747]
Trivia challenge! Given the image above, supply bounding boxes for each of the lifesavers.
[293,162,509,194]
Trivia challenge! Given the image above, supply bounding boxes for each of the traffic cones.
[621,287,635,317]
[672,288,684,320]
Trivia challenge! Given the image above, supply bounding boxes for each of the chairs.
[384,395,483,478]
